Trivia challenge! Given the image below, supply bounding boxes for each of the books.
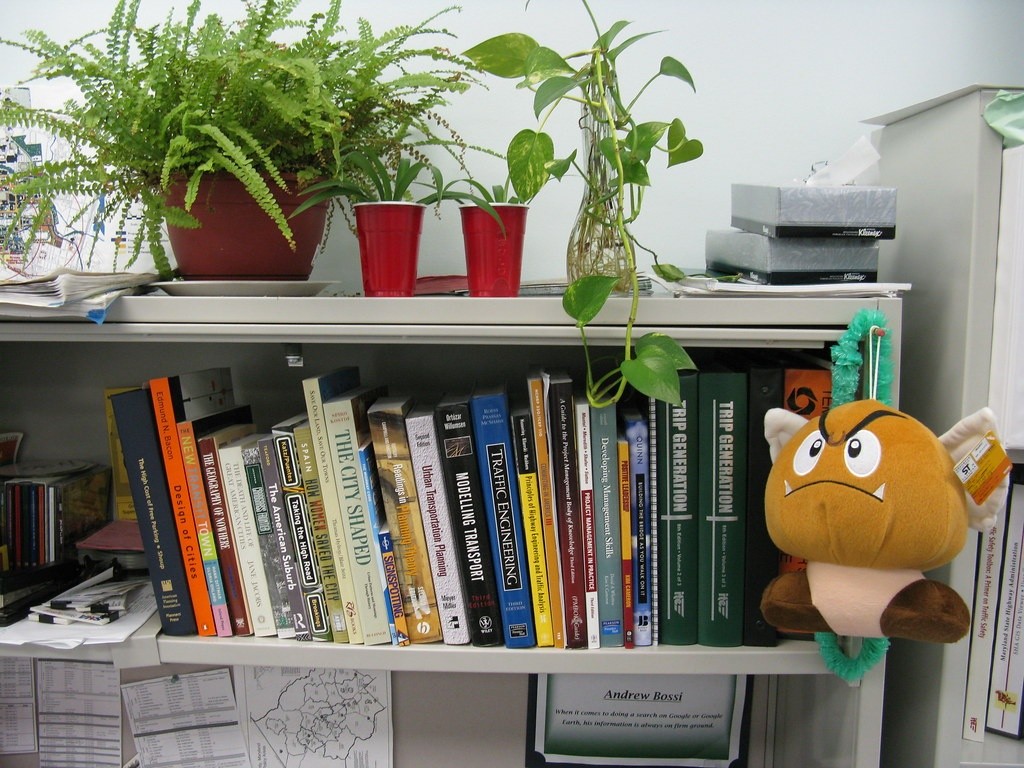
[962,468,1024,742]
[1,269,858,651]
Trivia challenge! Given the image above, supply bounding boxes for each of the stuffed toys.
[759,408,1011,644]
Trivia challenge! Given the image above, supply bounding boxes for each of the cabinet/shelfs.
[882,89,1024,768]
[0,281,912,768]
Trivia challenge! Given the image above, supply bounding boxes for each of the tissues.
[705,228,880,287]
[731,136,896,241]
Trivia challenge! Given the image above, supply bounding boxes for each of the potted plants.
[458,173,533,297]
[0,0,491,290]
[287,145,509,292]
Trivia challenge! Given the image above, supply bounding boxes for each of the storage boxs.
[703,228,881,286]
[731,182,898,242]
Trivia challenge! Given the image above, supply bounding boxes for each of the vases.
[565,94,637,299]
